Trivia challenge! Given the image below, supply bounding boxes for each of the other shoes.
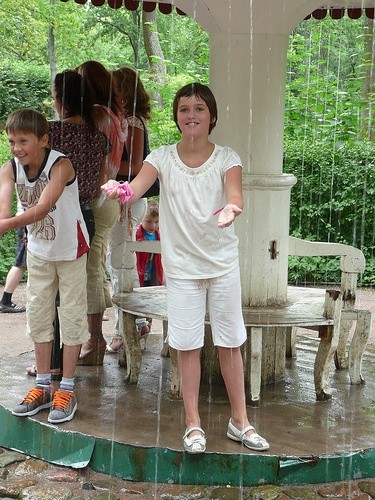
[226,418,270,451]
[182,426,207,455]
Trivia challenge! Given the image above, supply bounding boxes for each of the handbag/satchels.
[116,114,161,199]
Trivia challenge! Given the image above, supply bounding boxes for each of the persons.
[98,68,150,352]
[26,70,114,379]
[50,60,126,365]
[0,109,89,422]
[0,226,27,312]
[124,82,269,452]
[136,198,164,287]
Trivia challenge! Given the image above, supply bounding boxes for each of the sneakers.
[1,300,26,313]
[12,384,56,417]
[48,388,78,423]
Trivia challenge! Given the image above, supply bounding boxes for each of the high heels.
[138,321,150,350]
[105,335,124,354]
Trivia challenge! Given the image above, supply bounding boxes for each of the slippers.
[27,364,63,381]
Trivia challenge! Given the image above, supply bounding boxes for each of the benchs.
[113,284,371,403]
